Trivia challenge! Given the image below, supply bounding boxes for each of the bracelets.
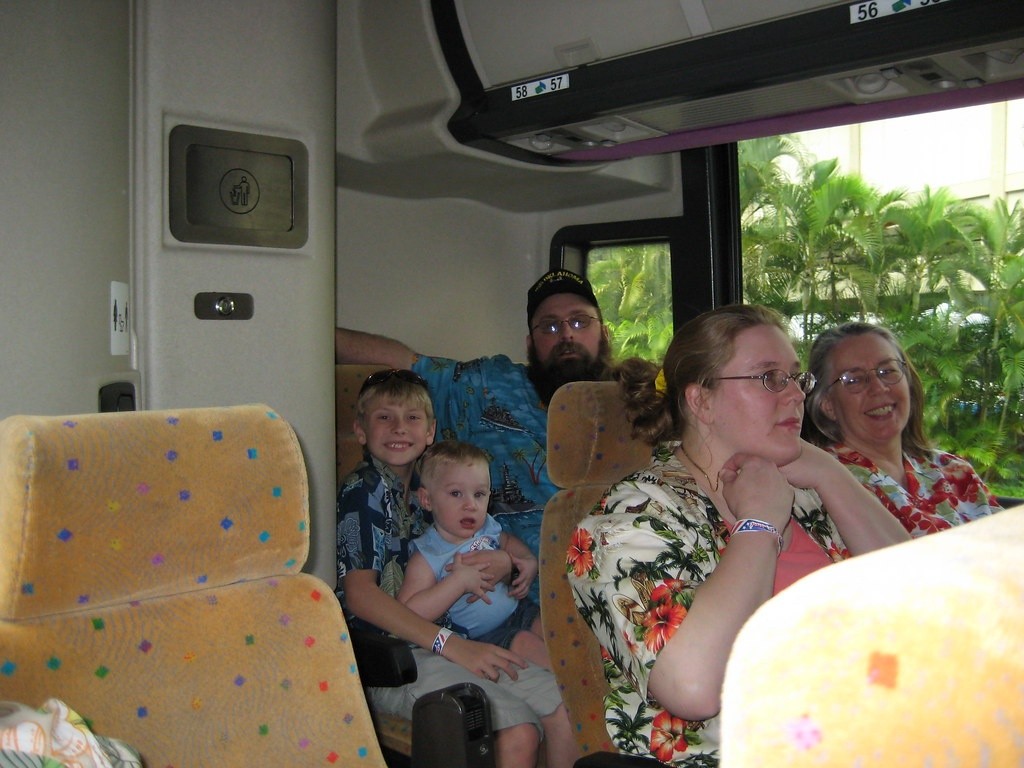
[731,517,783,557]
[432,628,453,656]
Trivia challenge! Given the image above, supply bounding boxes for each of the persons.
[565,307,909,768]
[809,322,1005,566]
[335,268,619,768]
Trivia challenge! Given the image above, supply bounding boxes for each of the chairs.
[718,502,1024,768]
[537,378,683,768]
[0,404,390,768]
[334,362,395,498]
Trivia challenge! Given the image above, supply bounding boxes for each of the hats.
[527,268,598,321]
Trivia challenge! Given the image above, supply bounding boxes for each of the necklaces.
[680,441,719,491]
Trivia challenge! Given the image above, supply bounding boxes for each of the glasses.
[709,369,817,393]
[359,369,427,396]
[832,360,906,394]
[533,314,599,334]
